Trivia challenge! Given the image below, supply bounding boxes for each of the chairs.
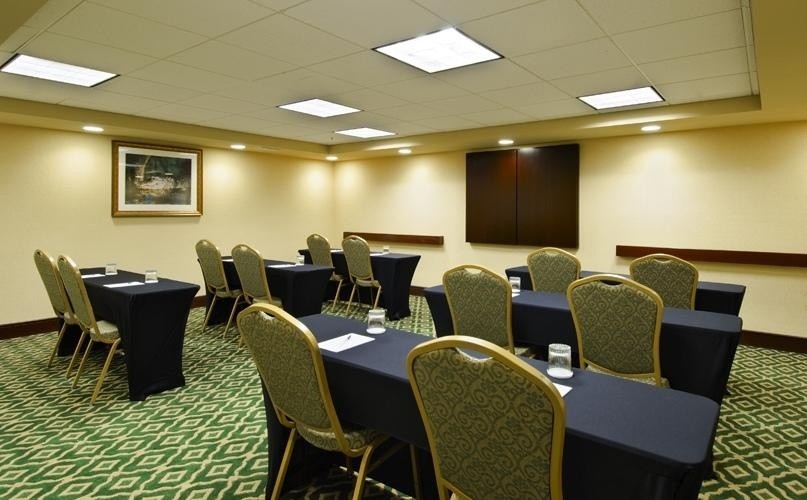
[34,248,89,380]
[57,255,123,406]
[443,263,516,355]
[230,244,282,349]
[566,272,672,389]
[342,234,391,314]
[526,247,582,294]
[305,234,361,315]
[629,253,698,311]
[237,302,421,498]
[406,336,568,500]
[195,239,248,342]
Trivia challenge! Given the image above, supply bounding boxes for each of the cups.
[105,262,119,276]
[509,276,520,294]
[384,245,390,254]
[367,309,385,333]
[145,270,158,283]
[296,255,306,266]
[547,342,571,379]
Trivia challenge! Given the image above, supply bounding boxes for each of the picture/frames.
[110,141,204,218]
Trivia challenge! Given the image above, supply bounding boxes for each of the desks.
[424,282,742,401]
[299,248,421,323]
[505,265,747,314]
[56,267,200,402]
[256,312,720,499]
[198,256,335,328]
